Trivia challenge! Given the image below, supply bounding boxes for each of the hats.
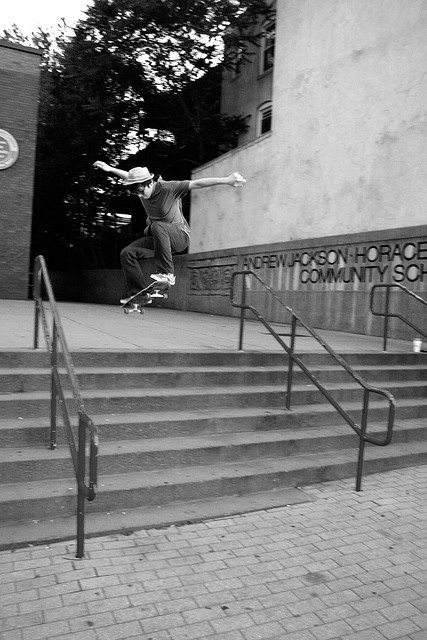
[122,166,154,185]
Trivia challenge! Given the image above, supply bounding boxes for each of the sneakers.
[150,273,176,286]
[120,294,152,304]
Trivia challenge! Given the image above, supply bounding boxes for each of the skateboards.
[120,281,170,314]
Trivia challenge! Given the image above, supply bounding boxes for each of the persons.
[93,160,246,306]
